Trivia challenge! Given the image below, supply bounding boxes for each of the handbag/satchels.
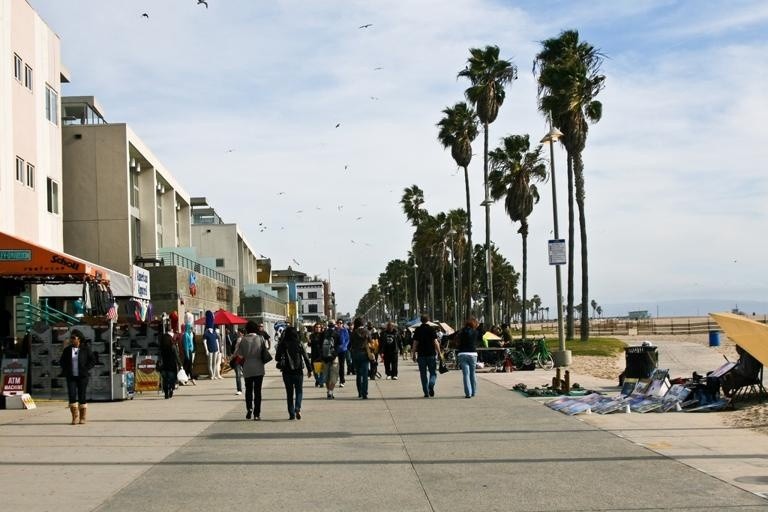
[365,342,376,361]
[261,338,272,364]
[439,360,447,374]
[230,356,241,369]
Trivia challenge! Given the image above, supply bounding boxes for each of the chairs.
[726,364,768,405]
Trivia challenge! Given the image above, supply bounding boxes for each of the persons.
[60,331,95,424]
[160,310,223,381]
[720,344,763,398]
[73,298,86,317]
[160,337,182,399]
[229,316,513,420]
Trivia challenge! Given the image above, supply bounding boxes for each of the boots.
[100,343,108,354]
[135,324,146,336]
[130,340,140,348]
[94,329,106,342]
[68,402,80,425]
[93,352,104,365]
[78,403,86,424]
[122,325,128,337]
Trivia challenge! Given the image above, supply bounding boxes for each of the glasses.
[70,336,78,340]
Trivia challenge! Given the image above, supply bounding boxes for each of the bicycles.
[504,335,556,371]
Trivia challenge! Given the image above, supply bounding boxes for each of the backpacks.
[321,331,335,363]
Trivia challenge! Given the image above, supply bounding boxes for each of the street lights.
[479,196,497,326]
[541,127,572,366]
[447,218,457,331]
[355,270,410,323]
[411,257,421,315]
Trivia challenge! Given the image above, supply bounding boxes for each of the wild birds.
[197,0,208,9]
[336,123,340,128]
[359,24,372,28]
[142,14,148,18]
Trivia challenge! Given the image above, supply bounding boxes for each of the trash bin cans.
[709,331,720,346]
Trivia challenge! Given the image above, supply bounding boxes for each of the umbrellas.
[195,307,248,354]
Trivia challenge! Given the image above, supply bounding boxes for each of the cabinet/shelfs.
[30,318,114,402]
[111,321,166,367]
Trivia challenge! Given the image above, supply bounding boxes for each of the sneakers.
[392,375,398,380]
[236,391,241,395]
[386,376,391,380]
[428,384,434,397]
[294,407,301,420]
[32,330,64,389]
[327,392,332,399]
[246,411,251,419]
[100,371,108,376]
[148,343,159,347]
